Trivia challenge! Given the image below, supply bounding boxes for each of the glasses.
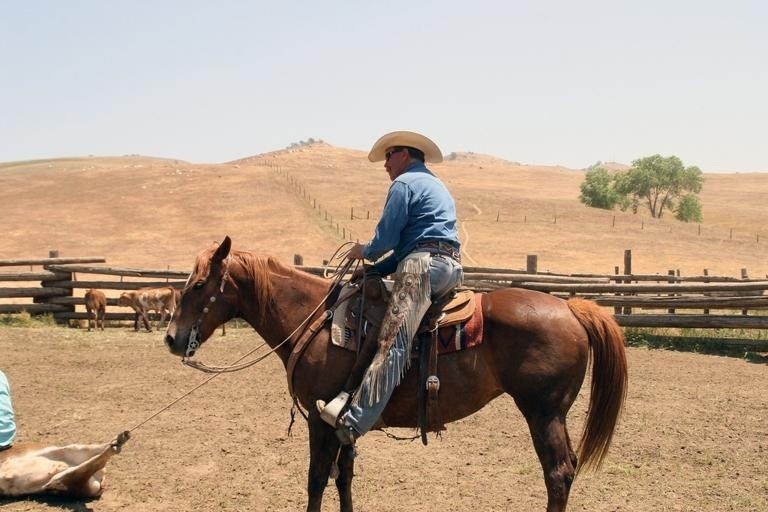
[385,150,401,160]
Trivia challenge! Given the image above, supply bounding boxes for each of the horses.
[164,235,628,512]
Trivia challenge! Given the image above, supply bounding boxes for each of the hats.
[369,132,442,165]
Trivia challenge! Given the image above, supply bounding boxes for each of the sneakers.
[314,397,357,445]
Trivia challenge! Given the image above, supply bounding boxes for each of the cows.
[84,286,184,332]
[0,429,132,500]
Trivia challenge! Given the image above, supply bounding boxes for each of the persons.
[0,371,17,450]
[315,130,463,446]
